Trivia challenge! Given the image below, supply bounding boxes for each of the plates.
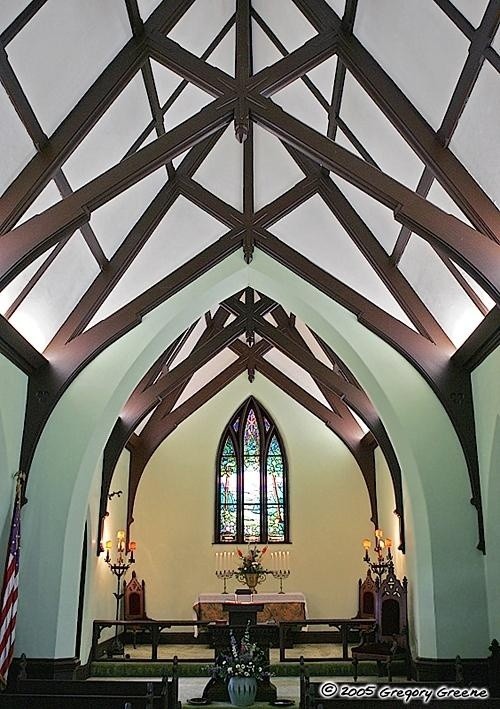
[188,697,210,705]
[271,700,295,706]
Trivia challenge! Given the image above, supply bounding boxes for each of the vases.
[235,566,268,596]
[225,674,259,707]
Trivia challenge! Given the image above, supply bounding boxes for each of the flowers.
[232,536,275,579]
[198,617,284,686]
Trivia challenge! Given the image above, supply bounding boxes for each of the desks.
[192,590,310,650]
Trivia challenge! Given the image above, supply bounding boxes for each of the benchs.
[0,651,184,709]
[296,649,500,709]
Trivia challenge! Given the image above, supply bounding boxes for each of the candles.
[212,550,237,577]
[269,549,292,576]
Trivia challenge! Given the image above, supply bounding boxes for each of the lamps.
[362,526,394,589]
[103,530,136,657]
